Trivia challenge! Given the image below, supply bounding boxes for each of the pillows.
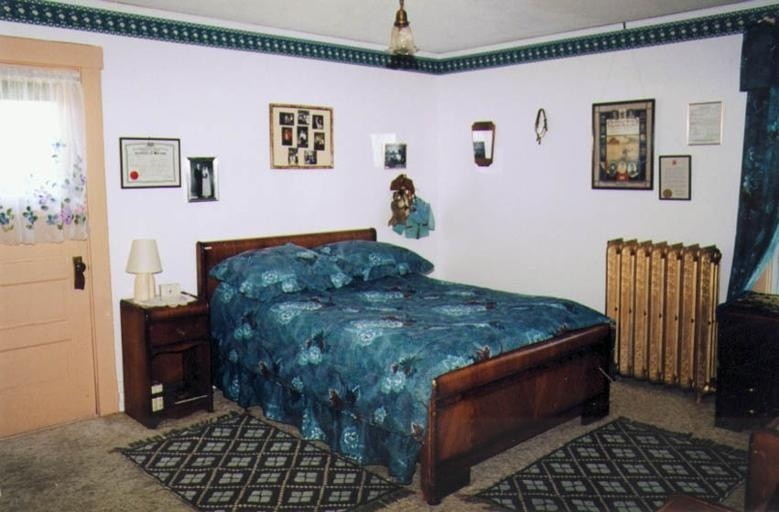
[312,239,435,282]
[208,242,352,303]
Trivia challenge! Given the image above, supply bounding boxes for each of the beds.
[196,228,613,505]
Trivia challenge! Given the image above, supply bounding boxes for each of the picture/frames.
[687,100,723,145]
[120,138,181,188]
[270,103,334,169]
[659,156,691,200]
[383,143,407,168]
[187,155,220,202]
[592,99,654,190]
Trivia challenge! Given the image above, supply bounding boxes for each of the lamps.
[388,0,417,55]
[125,239,161,301]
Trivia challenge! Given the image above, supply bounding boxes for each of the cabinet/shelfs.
[717,291,779,432]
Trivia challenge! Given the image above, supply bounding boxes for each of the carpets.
[474,416,748,512]
[108,411,416,512]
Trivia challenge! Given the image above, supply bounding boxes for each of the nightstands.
[121,291,213,429]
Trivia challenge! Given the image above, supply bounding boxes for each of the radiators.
[606,240,722,402]
